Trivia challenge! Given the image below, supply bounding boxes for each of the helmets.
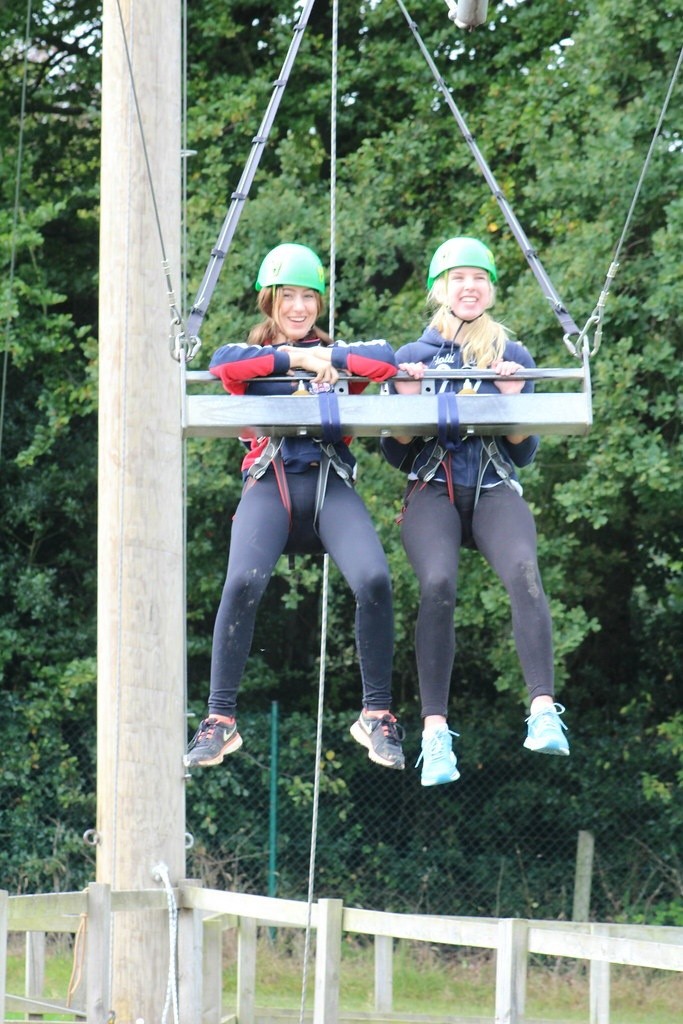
[427,237,497,290]
[256,242,325,295]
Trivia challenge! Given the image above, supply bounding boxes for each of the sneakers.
[183,717,243,767]
[350,709,406,770]
[415,724,461,786]
[523,703,570,755]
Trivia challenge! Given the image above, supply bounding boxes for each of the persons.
[393,233,573,791]
[178,245,407,773]
